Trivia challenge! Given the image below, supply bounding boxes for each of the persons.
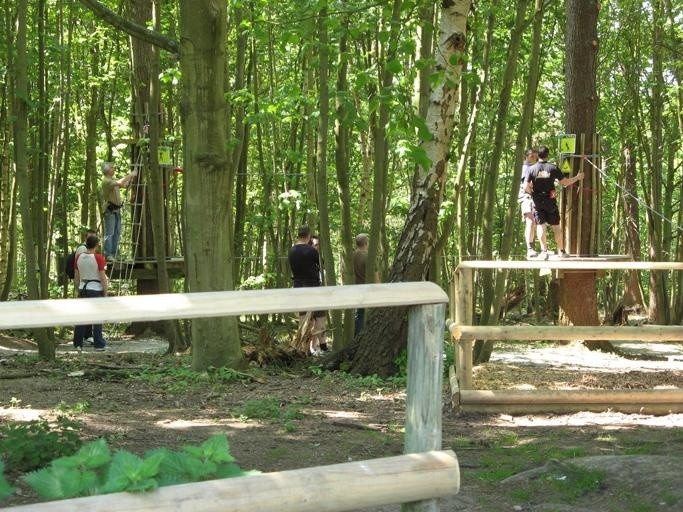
[522,145,585,260]
[99,160,138,261]
[306,234,323,358]
[73,228,99,343]
[287,226,333,356]
[350,232,381,340]
[73,234,109,348]
[516,148,554,260]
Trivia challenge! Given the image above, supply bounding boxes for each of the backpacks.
[65,243,88,282]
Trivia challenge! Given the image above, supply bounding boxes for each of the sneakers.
[527,247,571,261]
[306,349,333,359]
[105,253,126,262]
[69,338,112,351]
